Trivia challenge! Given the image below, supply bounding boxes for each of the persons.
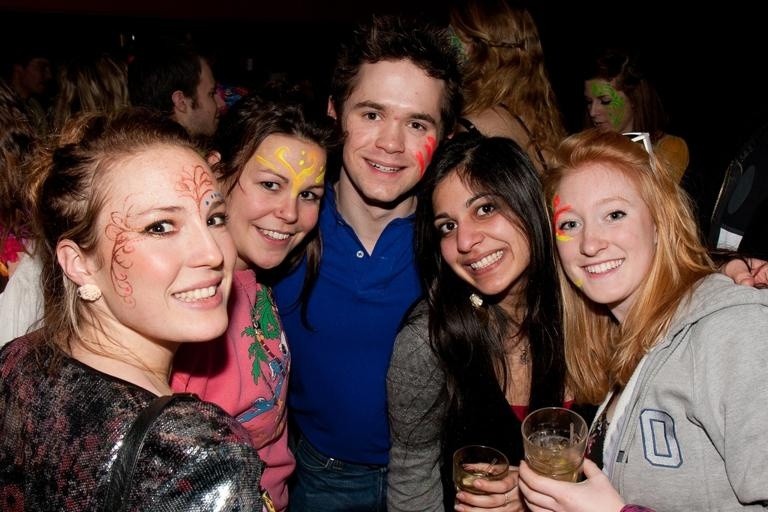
[0,1,768,511]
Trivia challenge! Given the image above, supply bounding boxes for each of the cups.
[519,407,591,485]
[452,443,509,505]
